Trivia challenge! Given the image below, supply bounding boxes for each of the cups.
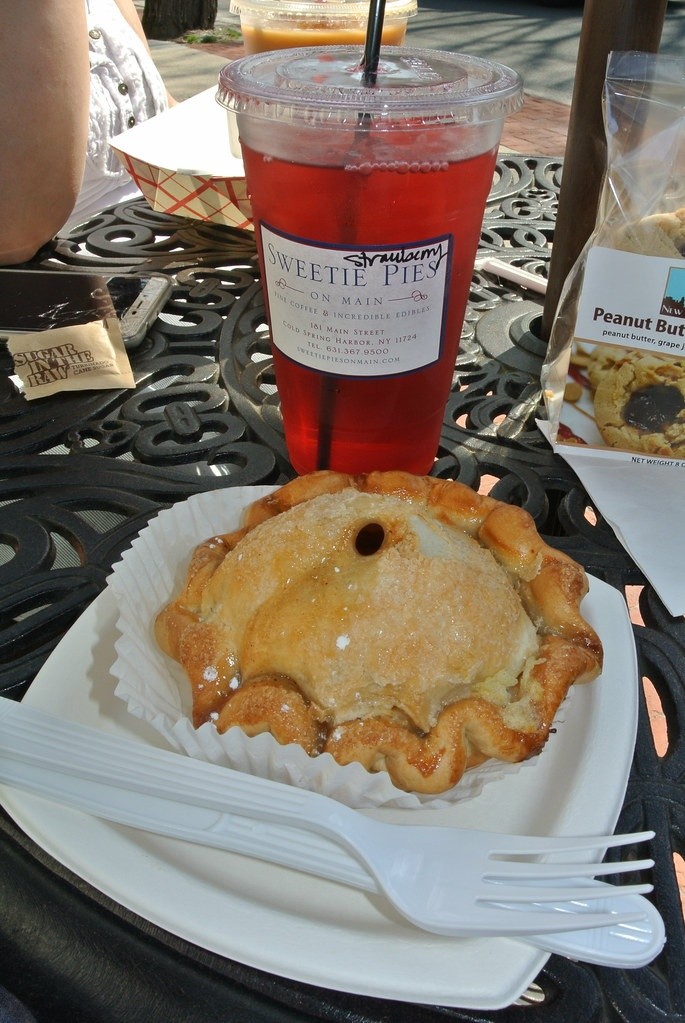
[229,0,417,55]
[219,46,523,476]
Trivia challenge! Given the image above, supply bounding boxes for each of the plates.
[0,568,666,1011]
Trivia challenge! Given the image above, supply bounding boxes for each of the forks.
[0,696,654,933]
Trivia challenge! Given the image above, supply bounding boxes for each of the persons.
[0,0,184,265]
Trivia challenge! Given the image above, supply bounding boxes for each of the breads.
[612,204,685,259]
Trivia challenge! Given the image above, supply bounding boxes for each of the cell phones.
[0,267,173,350]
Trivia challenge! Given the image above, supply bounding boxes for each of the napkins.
[533,418,685,617]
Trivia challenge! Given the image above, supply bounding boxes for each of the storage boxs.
[106,84,255,233]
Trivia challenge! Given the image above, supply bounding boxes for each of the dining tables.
[0,154,685,1023]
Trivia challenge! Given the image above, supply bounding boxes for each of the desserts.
[586,343,685,460]
[156,470,605,794]
